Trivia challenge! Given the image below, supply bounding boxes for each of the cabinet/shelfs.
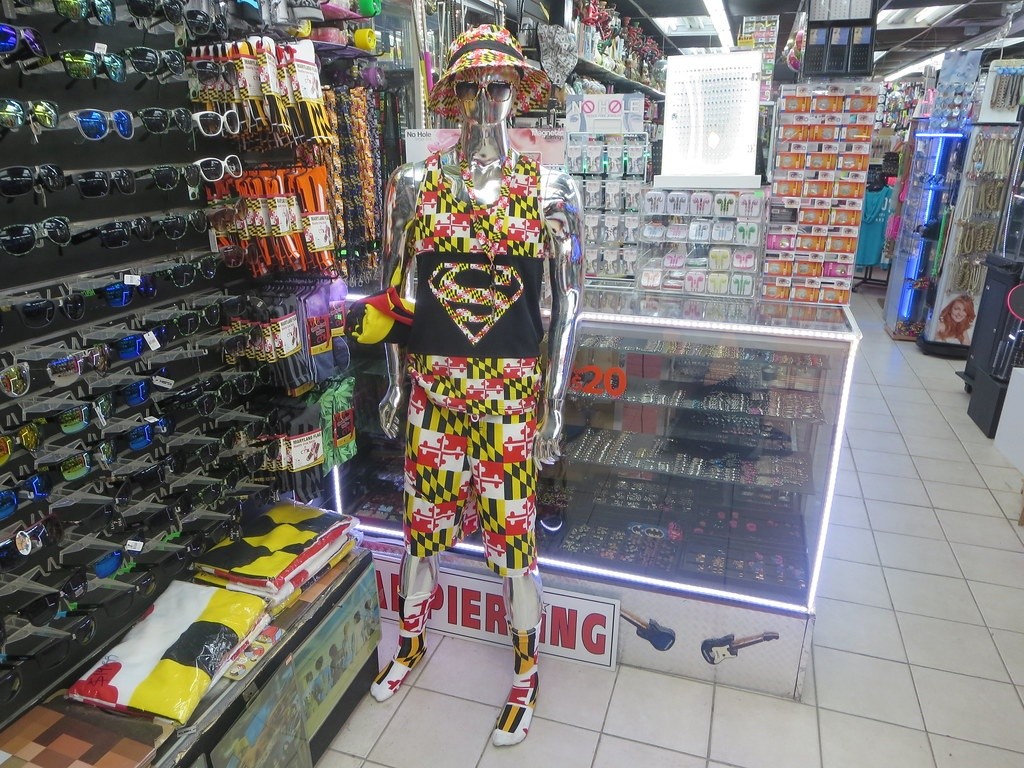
[328,288,865,702]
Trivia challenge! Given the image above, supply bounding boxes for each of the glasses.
[452,79,520,103]
[0,0,279,708]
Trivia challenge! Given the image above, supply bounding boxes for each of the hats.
[536,21,578,90]
[427,24,552,124]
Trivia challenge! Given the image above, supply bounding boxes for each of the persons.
[302,598,377,711]
[933,294,975,348]
[369,26,586,747]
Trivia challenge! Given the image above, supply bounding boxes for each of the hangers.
[867,170,889,192]
